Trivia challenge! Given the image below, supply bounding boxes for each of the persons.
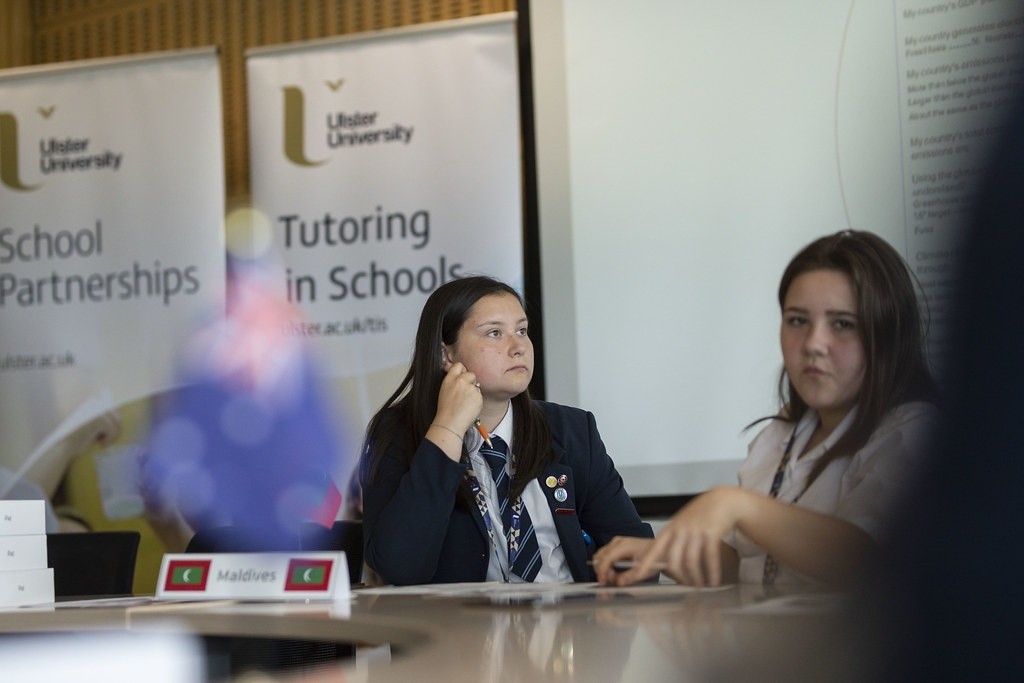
[593,227,937,599]
[359,277,658,595]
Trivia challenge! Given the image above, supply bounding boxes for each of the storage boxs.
[0,499,55,613]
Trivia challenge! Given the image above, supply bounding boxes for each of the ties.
[480,437,543,582]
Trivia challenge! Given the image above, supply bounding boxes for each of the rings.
[476,384,481,388]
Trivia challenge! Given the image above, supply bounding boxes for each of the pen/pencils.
[586,560,669,569]
[475,418,494,450]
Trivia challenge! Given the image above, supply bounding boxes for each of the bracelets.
[430,423,465,444]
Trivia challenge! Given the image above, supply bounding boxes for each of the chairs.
[181,517,367,595]
[45,531,141,600]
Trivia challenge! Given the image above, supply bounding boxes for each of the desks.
[0,578,963,683]
